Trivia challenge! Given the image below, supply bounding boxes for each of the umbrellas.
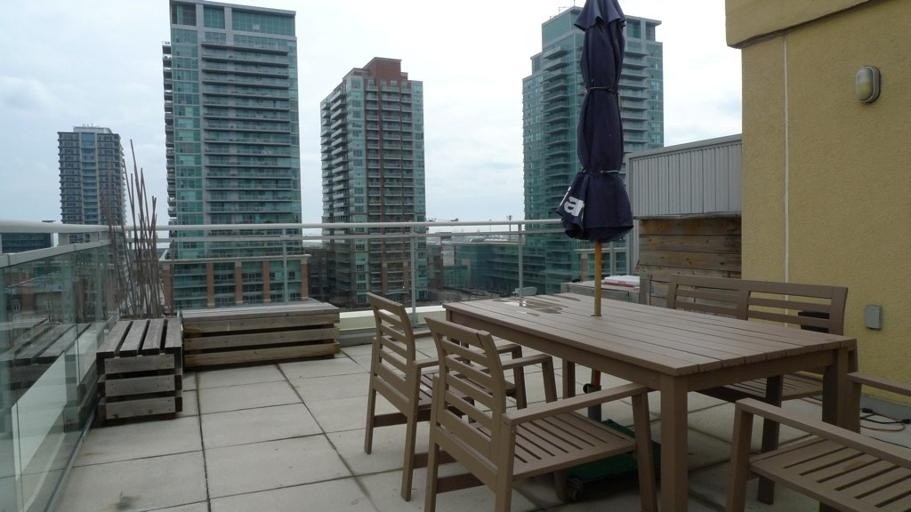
[558,0,635,388]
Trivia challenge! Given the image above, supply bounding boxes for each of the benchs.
[95,317,184,428]
[179,300,342,372]
